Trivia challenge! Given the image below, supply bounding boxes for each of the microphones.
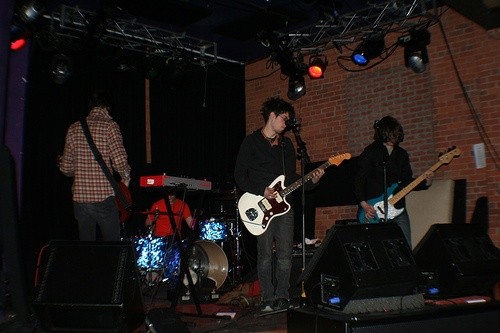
[285,119,296,125]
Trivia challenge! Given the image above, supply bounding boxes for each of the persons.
[234,98,325,313]
[60,91,132,240]
[357,116,434,249]
[145,187,194,238]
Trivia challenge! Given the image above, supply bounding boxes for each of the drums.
[135,213,234,292]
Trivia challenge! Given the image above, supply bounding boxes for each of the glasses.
[279,114,288,121]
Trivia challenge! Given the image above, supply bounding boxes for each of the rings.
[270,196,271,198]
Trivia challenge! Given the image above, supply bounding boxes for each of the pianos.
[139,175,212,314]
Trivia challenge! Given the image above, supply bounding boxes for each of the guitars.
[356,148,462,225]
[115,181,133,223]
[237,152,351,236]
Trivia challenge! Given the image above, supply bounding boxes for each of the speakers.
[295,221,421,310]
[412,222,500,301]
[32,238,144,330]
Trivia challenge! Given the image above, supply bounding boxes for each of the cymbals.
[209,213,235,216]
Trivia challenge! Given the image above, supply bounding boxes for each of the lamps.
[8,1,70,81]
[285,28,432,100]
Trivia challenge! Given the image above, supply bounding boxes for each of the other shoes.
[261,297,289,311]
